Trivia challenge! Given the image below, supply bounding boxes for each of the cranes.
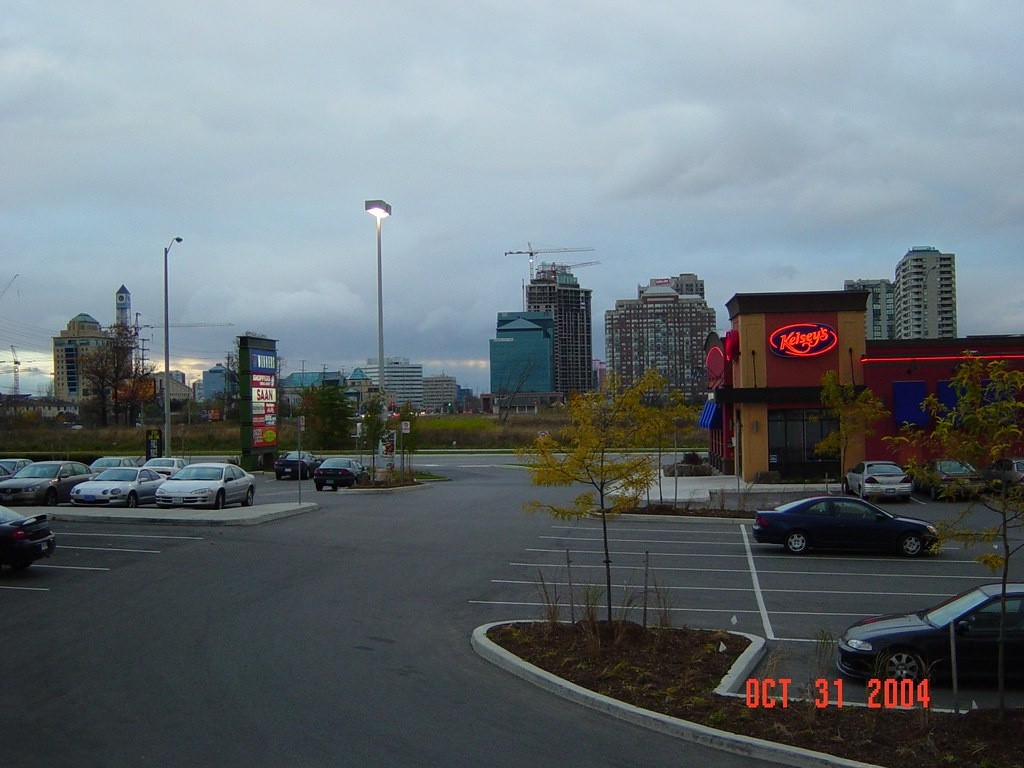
[10,345,21,394]
[534,258,602,276]
[100,322,236,340]
[503,242,597,277]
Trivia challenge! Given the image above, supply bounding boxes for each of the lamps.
[753,420,758,432]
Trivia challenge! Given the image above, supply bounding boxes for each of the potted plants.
[663,452,712,477]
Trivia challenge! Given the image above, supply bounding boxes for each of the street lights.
[364,198,394,417]
[163,235,185,459]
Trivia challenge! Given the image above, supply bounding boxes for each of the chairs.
[836,505,841,515]
[39,469,47,475]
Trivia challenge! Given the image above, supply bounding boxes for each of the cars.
[274,450,325,479]
[0,460,100,507]
[0,506,56,570]
[841,460,914,504]
[0,458,34,475]
[752,494,940,558]
[154,462,256,510]
[141,457,189,477]
[912,458,988,500]
[987,458,1024,496]
[835,583,1024,687]
[70,467,168,508]
[89,457,139,473]
[0,465,16,485]
[314,457,369,490]
